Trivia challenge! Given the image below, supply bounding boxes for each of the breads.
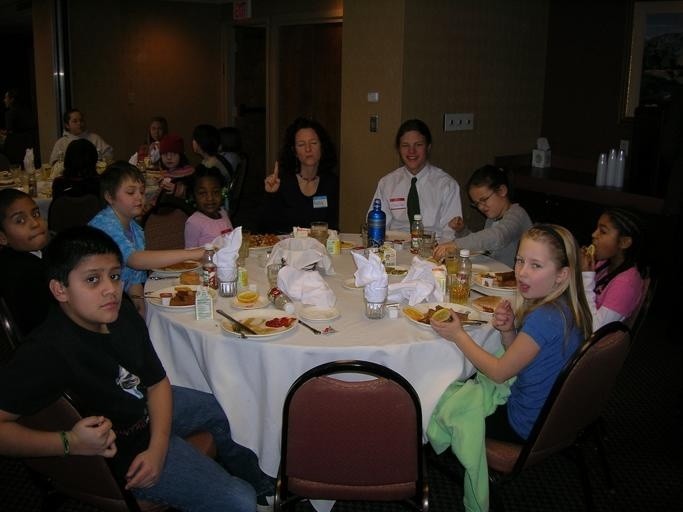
[471,295,504,313]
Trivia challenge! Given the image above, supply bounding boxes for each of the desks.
[506,162,664,217]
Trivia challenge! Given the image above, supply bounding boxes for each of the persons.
[180,167,236,250]
[0,188,80,338]
[256,119,339,230]
[82,158,224,313]
[429,221,595,443]
[0,107,248,238]
[431,163,536,272]
[576,206,652,340]
[0,221,282,511]
[365,119,465,243]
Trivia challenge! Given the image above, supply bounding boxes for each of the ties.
[407,177,420,232]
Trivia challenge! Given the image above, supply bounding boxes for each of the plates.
[151,259,200,273]
[472,271,517,293]
[299,305,339,320]
[471,305,493,316]
[469,253,480,257]
[146,284,216,309]
[341,278,364,291]
[406,302,480,329]
[219,295,299,337]
[248,245,273,249]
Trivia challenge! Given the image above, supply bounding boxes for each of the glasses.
[471,192,495,207]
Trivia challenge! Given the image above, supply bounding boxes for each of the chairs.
[274,360,425,511]
[8,373,210,511]
[483,322,632,508]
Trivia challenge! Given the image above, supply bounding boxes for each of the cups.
[443,247,461,291]
[267,263,285,290]
[217,265,237,297]
[311,221,327,248]
[360,223,367,248]
[449,275,469,304]
[363,285,388,319]
[419,229,436,257]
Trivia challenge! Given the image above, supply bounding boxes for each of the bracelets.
[59,430,70,458]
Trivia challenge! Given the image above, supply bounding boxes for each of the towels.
[264,237,335,273]
[349,248,390,309]
[275,266,336,309]
[211,226,245,282]
[386,255,448,306]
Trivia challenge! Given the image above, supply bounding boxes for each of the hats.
[159,131,184,153]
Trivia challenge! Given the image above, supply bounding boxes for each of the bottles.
[266,286,295,314]
[410,215,423,254]
[27,171,37,197]
[366,199,385,247]
[288,233,294,238]
[138,161,146,179]
[453,250,471,297]
[431,268,446,297]
[264,250,271,263]
[199,243,217,289]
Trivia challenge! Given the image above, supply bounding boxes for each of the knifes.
[215,309,256,335]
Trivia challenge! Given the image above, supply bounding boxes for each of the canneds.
[28,178,38,198]
